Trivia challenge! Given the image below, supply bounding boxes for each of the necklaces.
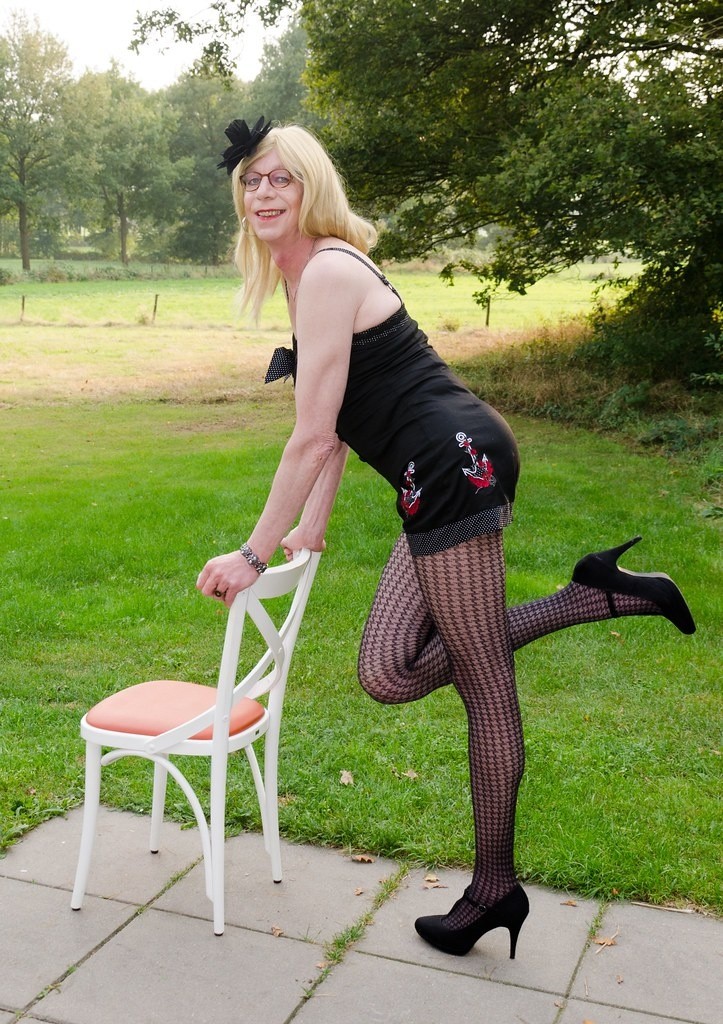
[288,235,319,304]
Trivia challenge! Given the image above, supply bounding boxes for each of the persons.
[195,115,698,960]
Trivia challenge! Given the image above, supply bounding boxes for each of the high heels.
[571,537,696,634]
[414,883,529,959]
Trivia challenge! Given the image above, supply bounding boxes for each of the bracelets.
[240,543,268,575]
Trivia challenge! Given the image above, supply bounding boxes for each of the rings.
[214,590,225,598]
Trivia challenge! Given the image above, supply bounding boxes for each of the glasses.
[240,168,293,192]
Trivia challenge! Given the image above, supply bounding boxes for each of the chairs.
[70,550,323,940]
